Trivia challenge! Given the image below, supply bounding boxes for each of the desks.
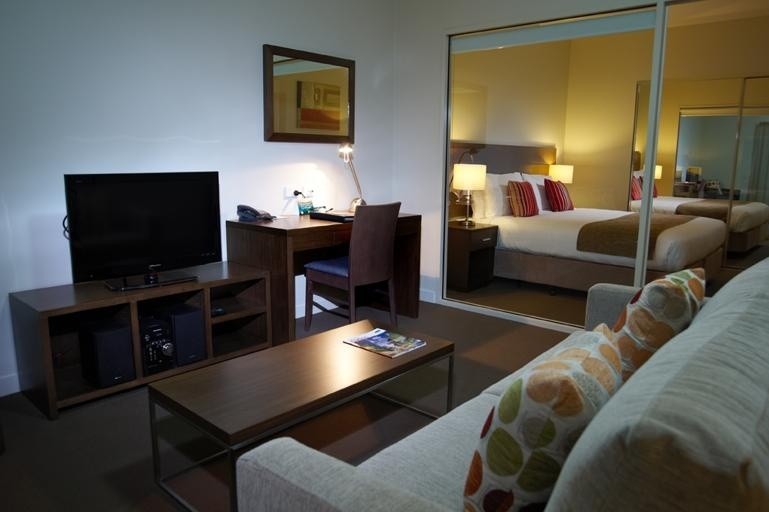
[226,198,422,348]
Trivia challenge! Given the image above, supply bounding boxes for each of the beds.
[628,151,769,254]
[448,141,726,292]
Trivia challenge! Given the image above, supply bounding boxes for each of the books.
[342,327,427,360]
[309,212,354,224]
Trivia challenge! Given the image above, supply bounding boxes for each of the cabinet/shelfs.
[9,261,272,422]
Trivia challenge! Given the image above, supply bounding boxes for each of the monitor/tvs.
[62,170,224,293]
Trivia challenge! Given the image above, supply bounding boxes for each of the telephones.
[236,204,277,223]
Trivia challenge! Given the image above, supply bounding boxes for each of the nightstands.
[448,220,497,297]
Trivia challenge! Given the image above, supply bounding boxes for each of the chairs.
[302,201,402,327]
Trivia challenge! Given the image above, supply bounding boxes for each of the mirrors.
[263,43,355,146]
[625,74,769,269]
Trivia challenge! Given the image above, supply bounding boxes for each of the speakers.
[77,320,135,389]
[154,303,203,369]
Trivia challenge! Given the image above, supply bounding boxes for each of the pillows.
[631,176,643,200]
[522,173,552,211]
[468,171,523,219]
[460,323,624,512]
[654,185,657,197]
[507,179,540,217]
[610,267,707,381]
[544,178,574,212]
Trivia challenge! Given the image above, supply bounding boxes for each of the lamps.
[338,142,367,213]
[655,165,662,179]
[453,163,487,226]
[549,164,574,185]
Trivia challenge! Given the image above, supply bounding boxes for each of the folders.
[308,209,355,224]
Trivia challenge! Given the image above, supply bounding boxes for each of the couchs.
[234,255,769,510]
